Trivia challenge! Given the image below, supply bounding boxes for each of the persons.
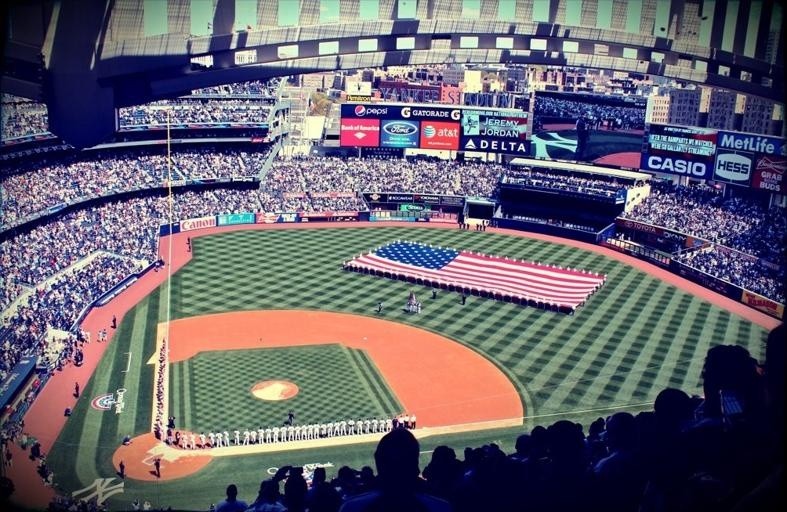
[0,60,787,510]
